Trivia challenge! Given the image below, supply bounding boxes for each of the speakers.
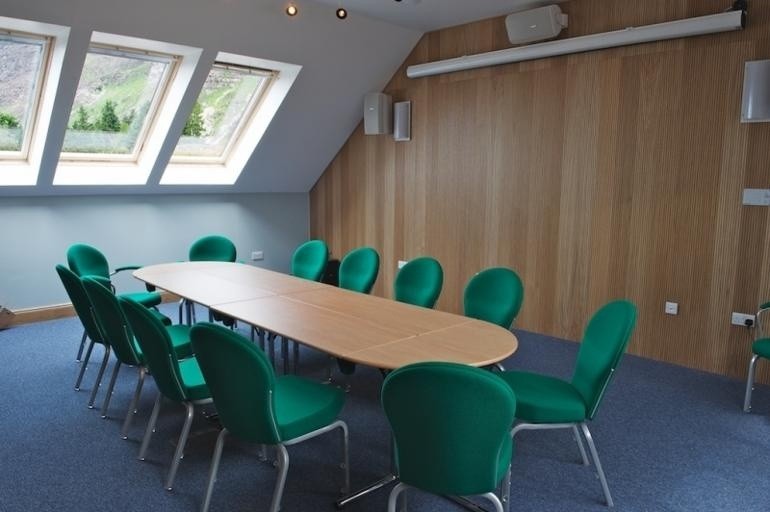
[363,92,392,134]
[504,5,567,45]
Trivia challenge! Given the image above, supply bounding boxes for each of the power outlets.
[731,313,755,327]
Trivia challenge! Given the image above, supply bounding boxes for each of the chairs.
[743,302,770,413]
